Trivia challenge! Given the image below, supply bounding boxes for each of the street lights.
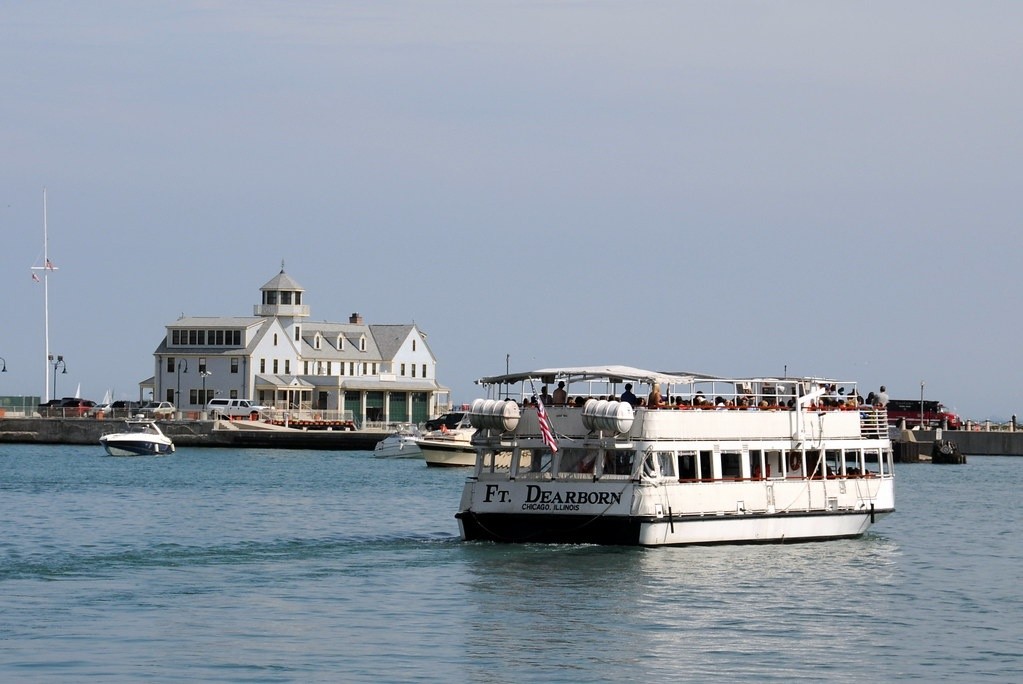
[47,354,68,400]
[175,358,189,412]
[919,380,927,431]
[200,370,212,413]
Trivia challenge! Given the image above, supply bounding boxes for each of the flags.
[32,274,40,283]
[47,259,53,273]
[529,377,559,455]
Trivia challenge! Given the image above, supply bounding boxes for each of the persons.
[814,463,870,480]
[504,381,889,413]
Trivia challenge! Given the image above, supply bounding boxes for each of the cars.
[94,403,112,418]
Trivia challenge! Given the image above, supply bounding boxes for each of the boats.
[453,353,899,551]
[98,417,177,457]
[412,422,532,469]
[372,424,424,460]
[211,427,399,452]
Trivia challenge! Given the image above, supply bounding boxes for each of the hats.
[698,397,707,403]
[558,381,565,387]
[625,383,632,390]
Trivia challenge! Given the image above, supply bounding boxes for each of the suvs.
[885,399,962,430]
[206,398,275,421]
[63,400,100,419]
[139,401,177,420]
[111,400,144,419]
[36,397,85,417]
[424,412,472,433]
